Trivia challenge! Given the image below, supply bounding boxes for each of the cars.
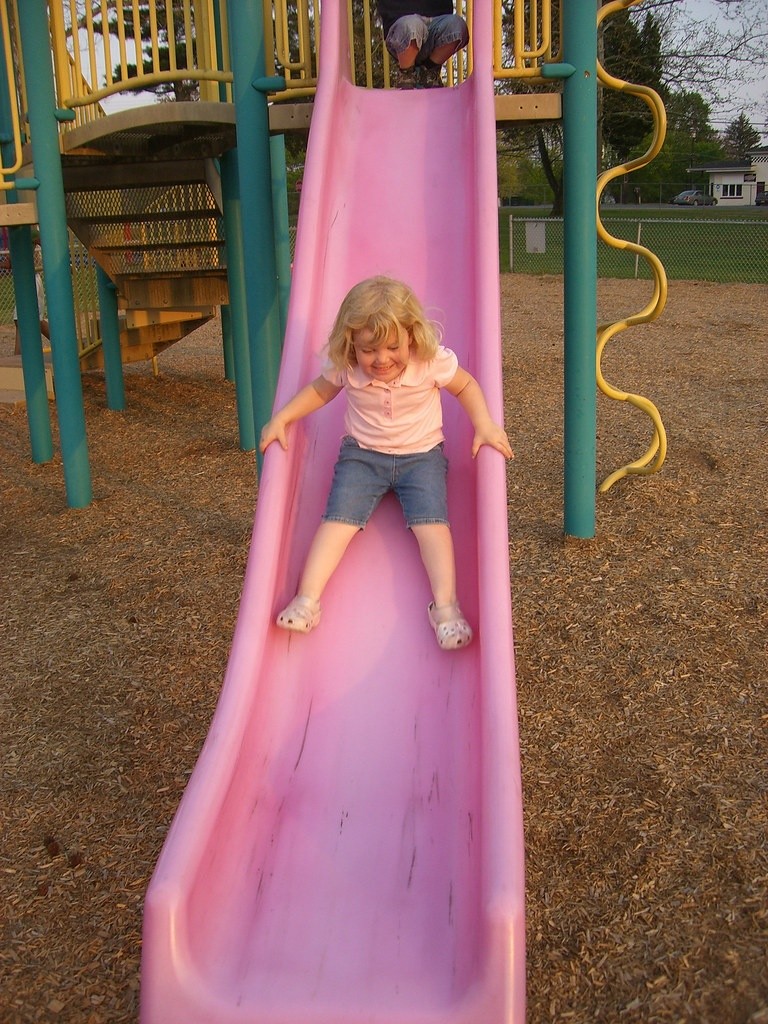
[755,190,768,206]
[673,189,718,206]
[601,194,617,206]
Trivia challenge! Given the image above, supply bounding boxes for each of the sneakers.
[395,64,444,90]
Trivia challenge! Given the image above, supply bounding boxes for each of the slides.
[142,0,531,1024]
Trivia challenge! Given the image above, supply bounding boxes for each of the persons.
[259,277,516,648]
[378,0,469,89]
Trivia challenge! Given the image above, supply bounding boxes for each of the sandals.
[276,595,321,633]
[428,600,472,650]
